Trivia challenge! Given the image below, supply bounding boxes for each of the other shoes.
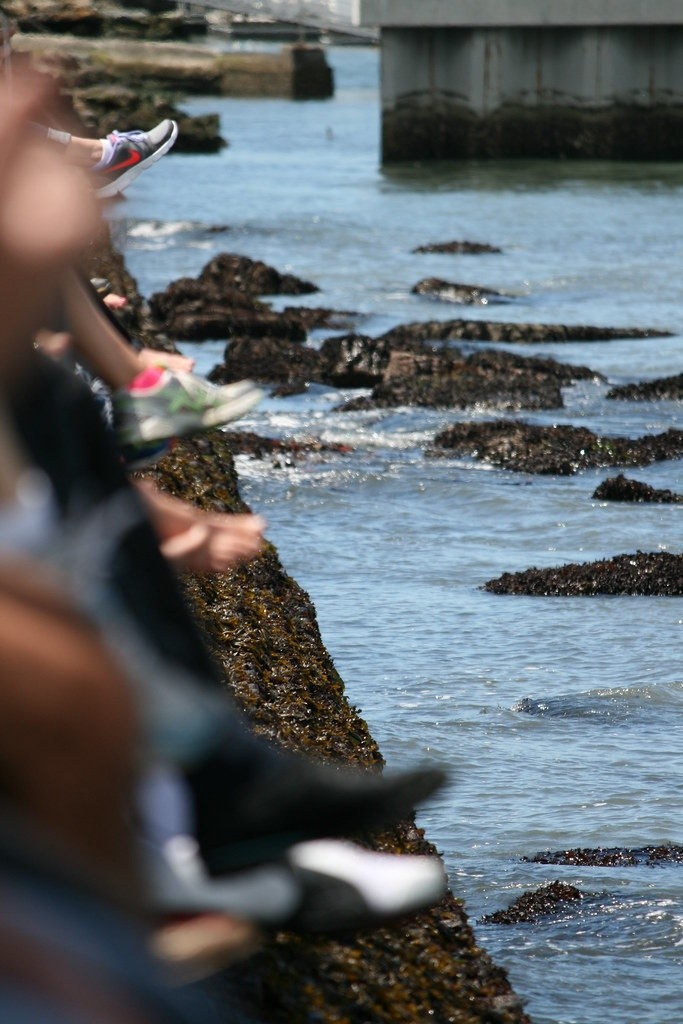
[289,833,447,916]
[195,742,453,879]
[267,860,370,938]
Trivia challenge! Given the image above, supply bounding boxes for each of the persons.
[0,32,448,1023]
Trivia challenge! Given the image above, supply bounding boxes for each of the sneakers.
[88,118,178,200]
[112,370,266,467]
